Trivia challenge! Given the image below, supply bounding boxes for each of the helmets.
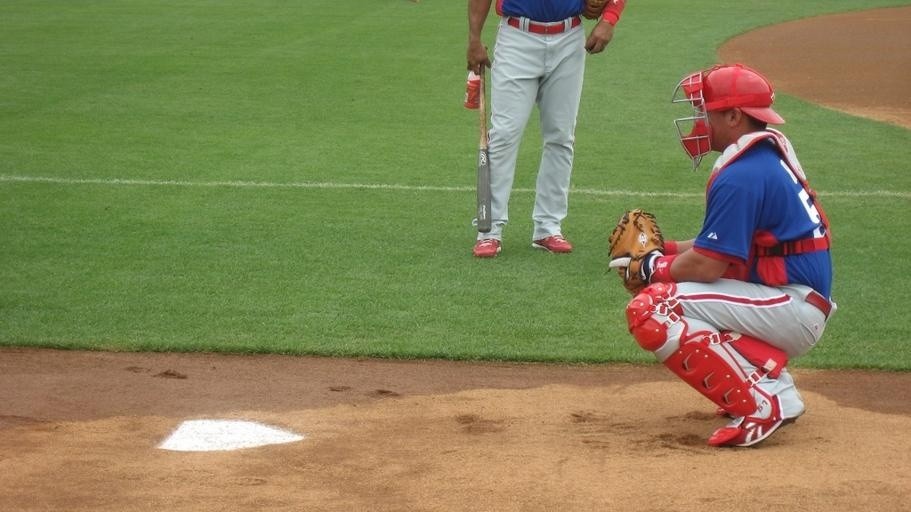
[671,63,786,172]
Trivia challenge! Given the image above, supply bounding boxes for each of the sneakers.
[708,385,806,446]
[472,239,501,258]
[533,235,572,254]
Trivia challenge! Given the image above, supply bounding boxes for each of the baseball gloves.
[608,209,663,291]
[582,0,607,19]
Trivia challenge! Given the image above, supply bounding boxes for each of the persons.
[605,62,839,450]
[462,1,627,259]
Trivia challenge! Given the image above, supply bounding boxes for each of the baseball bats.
[477,45,491,232]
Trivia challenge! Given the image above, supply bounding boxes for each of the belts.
[508,16,582,34]
[805,292,832,318]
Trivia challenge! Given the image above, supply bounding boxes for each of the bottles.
[463,71,481,112]
